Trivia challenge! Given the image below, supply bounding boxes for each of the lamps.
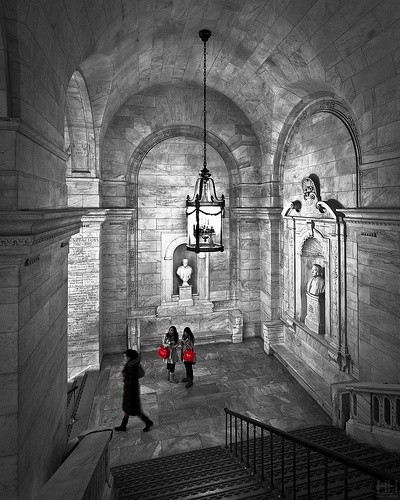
[186,29,225,254]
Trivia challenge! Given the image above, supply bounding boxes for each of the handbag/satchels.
[158,345,170,359]
[183,347,194,362]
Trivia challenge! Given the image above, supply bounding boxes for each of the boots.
[141,414,153,431]
[114,416,129,432]
[166,368,176,383]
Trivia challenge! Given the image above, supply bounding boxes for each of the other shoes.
[187,382,193,388]
[183,378,188,381]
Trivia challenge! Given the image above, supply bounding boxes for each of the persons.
[115,349,154,432]
[181,327,195,389]
[306,263,324,296]
[176,259,194,281]
[162,326,180,384]
